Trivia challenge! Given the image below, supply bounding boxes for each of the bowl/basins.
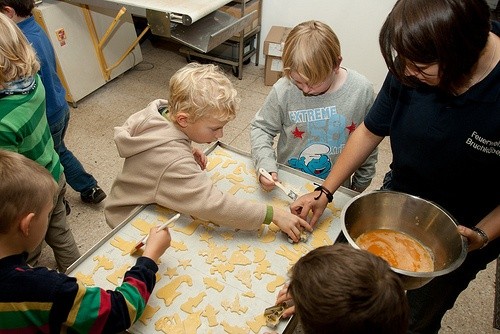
[341,190,469,290]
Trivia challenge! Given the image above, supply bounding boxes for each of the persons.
[290,1,500,334]
[0,13,80,274]
[103,63,315,243]
[0,152,171,333]
[250,20,379,194]
[0,0,106,215]
[276,244,410,334]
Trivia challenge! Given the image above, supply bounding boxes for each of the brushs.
[130,213,181,256]
[259,167,300,201]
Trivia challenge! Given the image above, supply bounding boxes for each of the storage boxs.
[263,25,294,57]
[264,55,283,86]
[218,0,258,37]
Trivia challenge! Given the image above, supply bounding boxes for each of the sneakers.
[81,187,106,203]
[63,200,71,216]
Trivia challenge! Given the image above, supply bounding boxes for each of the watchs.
[470,225,490,249]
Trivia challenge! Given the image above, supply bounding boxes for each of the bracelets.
[313,184,334,204]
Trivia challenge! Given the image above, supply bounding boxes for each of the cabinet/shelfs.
[179,0,263,80]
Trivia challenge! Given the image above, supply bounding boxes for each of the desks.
[32,0,143,108]
